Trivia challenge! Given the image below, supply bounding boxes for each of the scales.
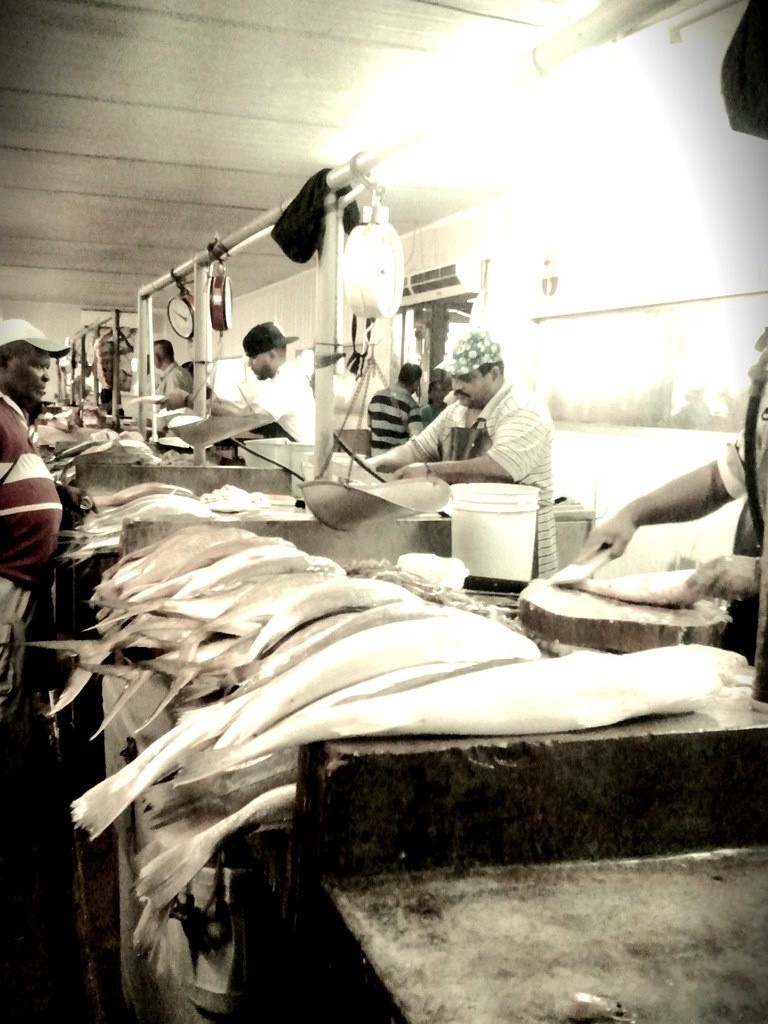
[155,277,195,424]
[298,191,455,531]
[166,261,277,448]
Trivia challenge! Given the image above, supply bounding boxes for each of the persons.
[153,339,216,399]
[574,328,768,668]
[367,330,558,581]
[0,318,87,731]
[368,364,423,458]
[421,381,448,426]
[155,322,316,444]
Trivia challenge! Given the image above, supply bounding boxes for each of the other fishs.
[0,481,755,962]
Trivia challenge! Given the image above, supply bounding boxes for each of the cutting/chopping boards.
[516,582,731,652]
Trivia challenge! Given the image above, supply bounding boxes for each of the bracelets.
[755,557,761,588]
[184,396,188,405]
[425,463,431,475]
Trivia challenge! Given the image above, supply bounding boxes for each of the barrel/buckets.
[242,435,315,499]
[333,429,372,461]
[449,481,539,592]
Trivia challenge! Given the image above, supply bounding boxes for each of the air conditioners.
[398,258,479,308]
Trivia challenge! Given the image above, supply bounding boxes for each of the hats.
[434,326,501,376]
[0,318,70,359]
[243,322,298,357]
